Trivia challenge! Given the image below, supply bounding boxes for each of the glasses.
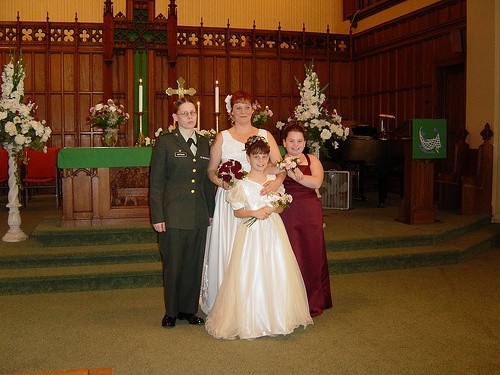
[176,111,196,117]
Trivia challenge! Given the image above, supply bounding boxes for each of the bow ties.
[187,138,197,148]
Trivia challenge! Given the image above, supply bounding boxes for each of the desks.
[59,146,152,221]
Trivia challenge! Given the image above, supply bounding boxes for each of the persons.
[204,136,314,339]
[202,90,287,317]
[150,97,215,329]
[277,124,334,317]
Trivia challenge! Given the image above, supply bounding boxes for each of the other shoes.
[311,309,323,317]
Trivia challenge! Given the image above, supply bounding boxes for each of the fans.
[319,168,353,209]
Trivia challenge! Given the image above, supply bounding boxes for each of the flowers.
[197,128,217,146]
[146,125,178,145]
[225,94,232,112]
[244,192,293,227]
[0,47,52,187]
[275,60,351,154]
[86,99,130,125]
[278,156,303,180]
[251,100,272,127]
[218,158,249,187]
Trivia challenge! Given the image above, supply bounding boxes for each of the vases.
[2,141,28,242]
[102,125,118,146]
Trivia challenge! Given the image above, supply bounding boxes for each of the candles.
[138,79,144,112]
[214,81,219,113]
[197,101,201,130]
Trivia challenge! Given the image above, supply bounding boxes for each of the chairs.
[0,146,61,208]
[438,123,492,213]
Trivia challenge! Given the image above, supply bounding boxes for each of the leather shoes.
[162,315,176,327]
[177,312,204,324]
